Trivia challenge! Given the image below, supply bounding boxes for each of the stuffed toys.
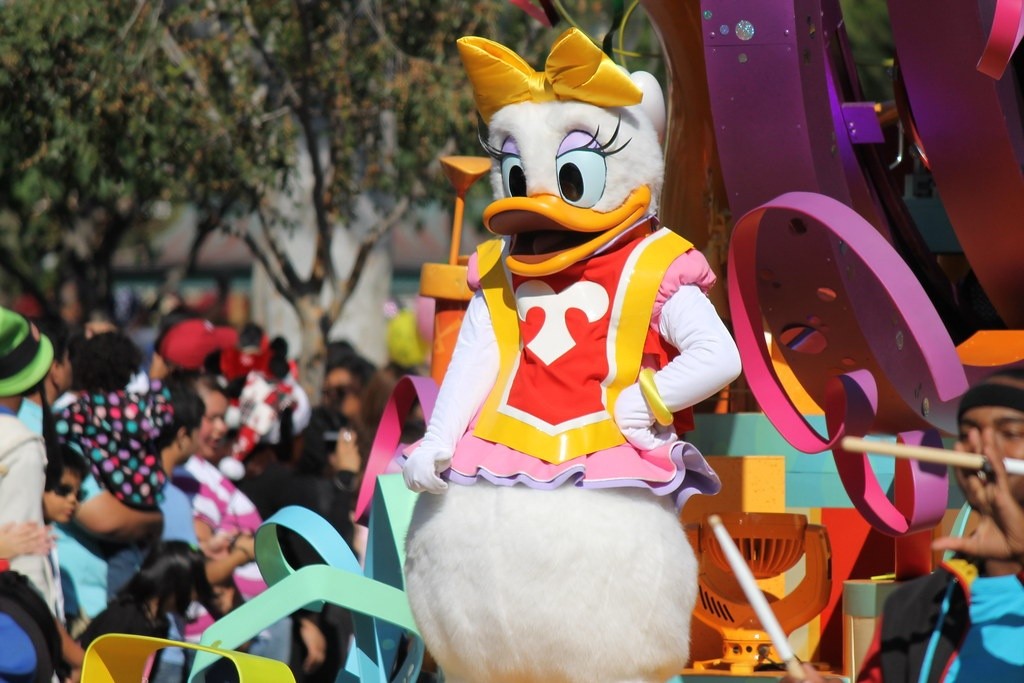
[398,25,743,683]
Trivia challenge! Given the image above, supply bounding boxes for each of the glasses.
[48,483,88,502]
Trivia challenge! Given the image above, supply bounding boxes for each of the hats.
[159,319,239,369]
[0,306,54,397]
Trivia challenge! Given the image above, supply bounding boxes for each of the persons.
[0,274,439,683]
[781,361,1024,683]
